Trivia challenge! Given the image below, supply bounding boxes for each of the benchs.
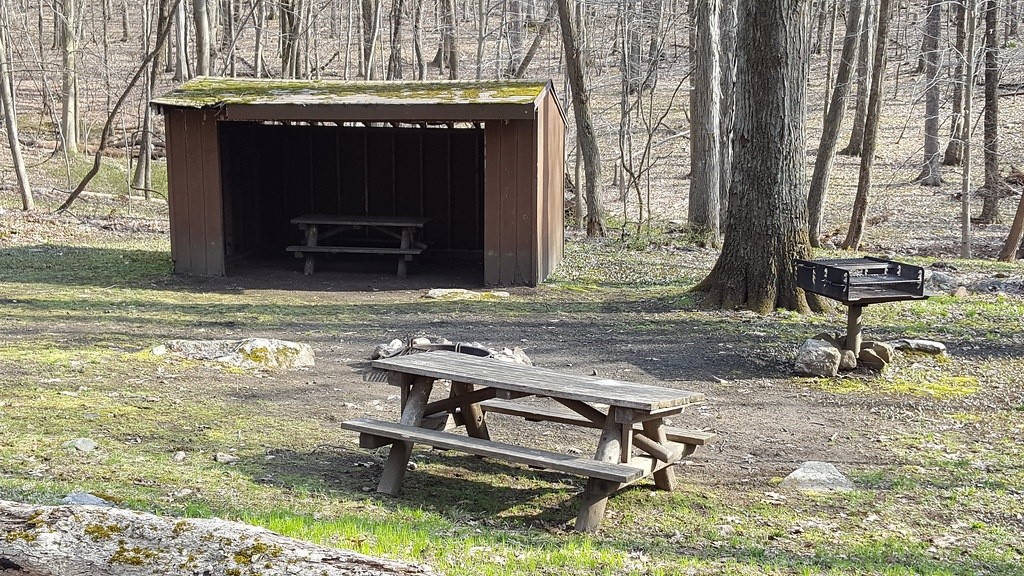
[477,399,717,445]
[340,416,644,483]
[286,238,436,255]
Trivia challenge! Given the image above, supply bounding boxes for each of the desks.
[340,350,716,534]
[286,212,436,279]
